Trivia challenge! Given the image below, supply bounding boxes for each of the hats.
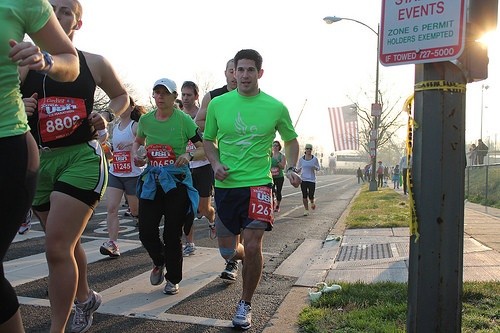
[153,78,177,94]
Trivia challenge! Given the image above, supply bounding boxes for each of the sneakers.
[209,224,217,240]
[163,280,179,295]
[71,289,102,333]
[100,240,120,257]
[220,260,239,280]
[197,213,202,219]
[122,201,129,207]
[232,300,251,330]
[18,210,33,235]
[182,242,197,257]
[150,262,165,285]
[125,207,131,216]
[133,217,139,226]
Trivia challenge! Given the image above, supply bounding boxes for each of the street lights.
[322,14,380,192]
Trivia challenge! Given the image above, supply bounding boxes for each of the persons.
[19,210,33,235]
[18,0,130,333]
[296,144,320,215]
[473,139,488,168]
[203,49,302,329]
[470,144,477,169]
[364,164,371,182]
[100,96,146,257]
[377,161,384,187]
[357,167,364,184]
[130,59,244,281]
[0,0,80,333]
[270,141,286,212]
[400,155,413,194]
[132,78,207,295]
[392,165,400,189]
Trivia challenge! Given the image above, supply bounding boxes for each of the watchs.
[187,152,194,161]
[41,50,54,74]
[105,110,114,122]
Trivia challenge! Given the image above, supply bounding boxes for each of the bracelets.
[287,167,298,174]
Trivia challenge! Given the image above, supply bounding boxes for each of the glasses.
[306,148,312,150]
[273,145,279,147]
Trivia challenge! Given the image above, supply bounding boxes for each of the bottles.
[101,142,114,160]
[136,144,148,170]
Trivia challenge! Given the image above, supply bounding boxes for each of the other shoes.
[304,209,309,215]
[274,206,280,212]
[311,202,316,210]
[273,201,276,210]
